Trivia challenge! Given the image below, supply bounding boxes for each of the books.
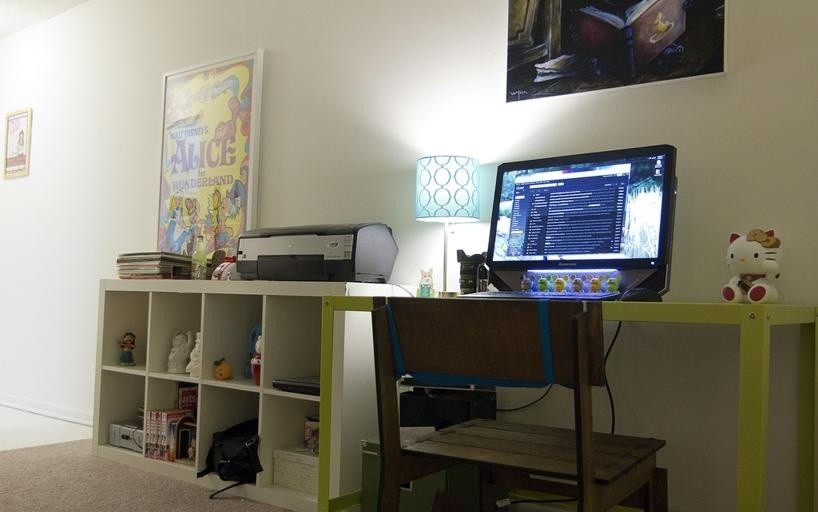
[301,413,320,446]
[145,407,194,465]
[114,250,195,280]
[175,380,198,410]
[270,446,319,500]
[573,0,687,81]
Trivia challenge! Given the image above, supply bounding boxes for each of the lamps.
[415,156,480,297]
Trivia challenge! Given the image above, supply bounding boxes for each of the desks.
[318,295,817,512]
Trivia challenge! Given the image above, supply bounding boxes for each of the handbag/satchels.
[204,418,264,484]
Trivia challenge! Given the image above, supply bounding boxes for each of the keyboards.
[457,289,620,299]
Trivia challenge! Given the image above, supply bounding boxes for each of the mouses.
[623,287,662,302]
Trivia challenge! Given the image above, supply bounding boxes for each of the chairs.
[372,297,666,512]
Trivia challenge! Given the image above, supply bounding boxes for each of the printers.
[237,222,399,283]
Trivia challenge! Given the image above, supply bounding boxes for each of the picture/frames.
[156,47,264,264]
[3,108,32,178]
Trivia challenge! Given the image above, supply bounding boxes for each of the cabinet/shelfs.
[92,279,418,512]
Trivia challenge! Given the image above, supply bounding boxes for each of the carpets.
[0,439,294,512]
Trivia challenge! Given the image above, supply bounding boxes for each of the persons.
[561,1,697,39]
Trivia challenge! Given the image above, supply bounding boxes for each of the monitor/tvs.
[486,143,676,300]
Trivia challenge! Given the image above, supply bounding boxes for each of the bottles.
[477,258,489,292]
[190,235,206,280]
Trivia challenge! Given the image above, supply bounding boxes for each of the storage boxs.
[360,441,468,512]
[272,447,319,496]
